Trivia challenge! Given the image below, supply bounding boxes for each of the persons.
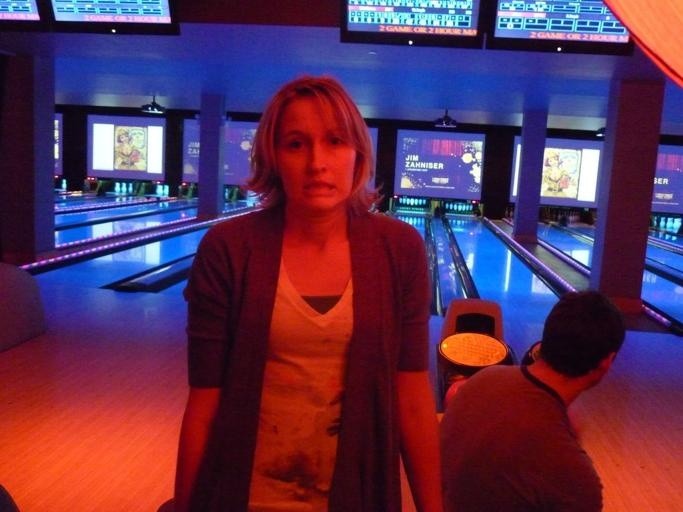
[156,76,443,512]
[438,289,625,511]
[543,150,570,197]
[114,128,143,169]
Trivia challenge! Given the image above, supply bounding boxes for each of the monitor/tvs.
[0,0,45,31]
[47,0,181,36]
[486,0,636,57]
[339,0,486,50]
